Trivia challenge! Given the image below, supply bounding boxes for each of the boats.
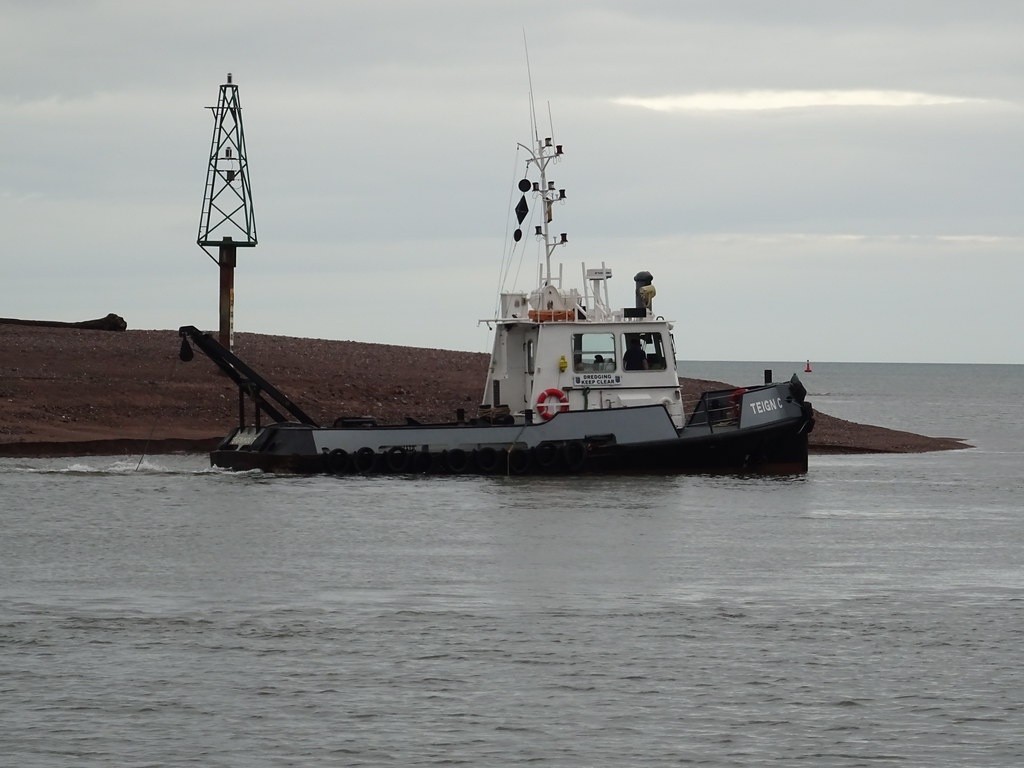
[179,25,815,477]
[528,310,576,322]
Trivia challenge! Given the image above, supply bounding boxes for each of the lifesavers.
[536,388,569,421]
[351,447,377,474]
[386,445,409,472]
[508,449,530,474]
[324,448,353,476]
[728,388,750,417]
[447,448,468,474]
[476,447,499,472]
[536,443,558,469]
[528,310,574,321]
[566,440,587,464]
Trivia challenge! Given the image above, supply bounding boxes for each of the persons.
[623,338,648,370]
[594,355,605,363]
